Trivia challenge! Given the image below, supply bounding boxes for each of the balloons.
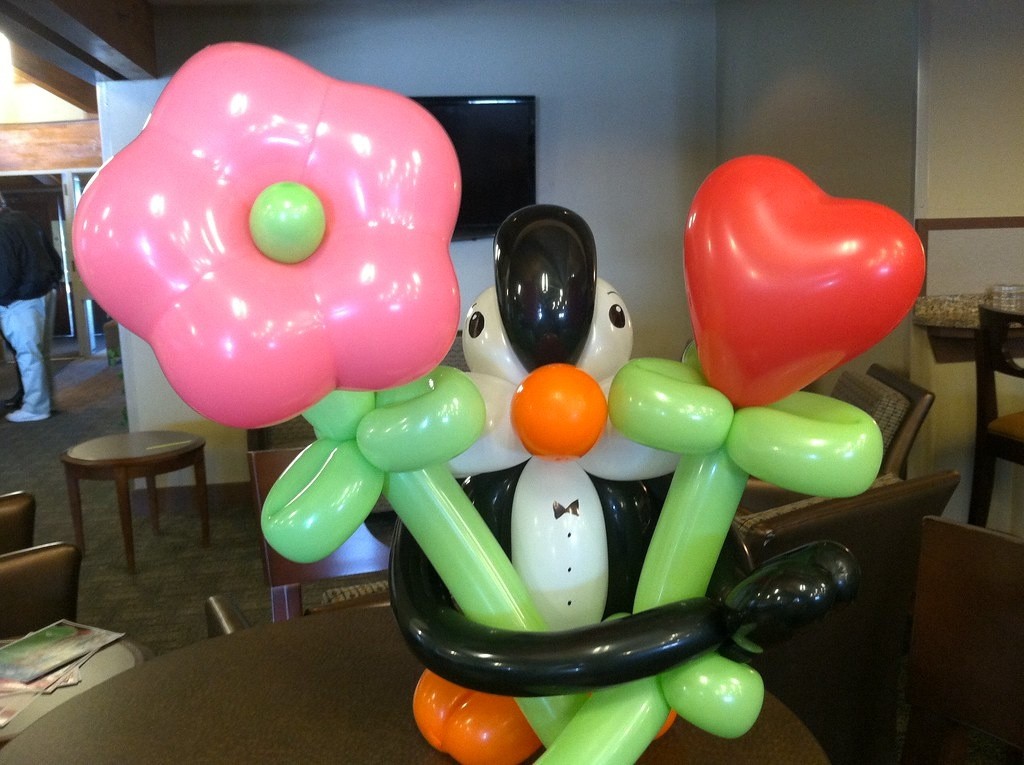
[71,41,925,765]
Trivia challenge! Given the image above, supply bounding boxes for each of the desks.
[58,429,212,571]
[0,602,831,765]
[0,629,146,748]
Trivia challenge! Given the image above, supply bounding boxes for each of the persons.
[0,198,55,422]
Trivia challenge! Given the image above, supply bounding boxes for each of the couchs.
[205,572,390,638]
[0,489,82,639]
[703,361,963,764]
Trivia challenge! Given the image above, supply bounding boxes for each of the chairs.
[966,303,1024,527]
[246,446,393,622]
[896,512,1024,765]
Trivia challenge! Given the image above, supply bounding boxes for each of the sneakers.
[5,410,52,423]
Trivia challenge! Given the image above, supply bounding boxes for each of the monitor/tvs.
[407,95,537,237]
